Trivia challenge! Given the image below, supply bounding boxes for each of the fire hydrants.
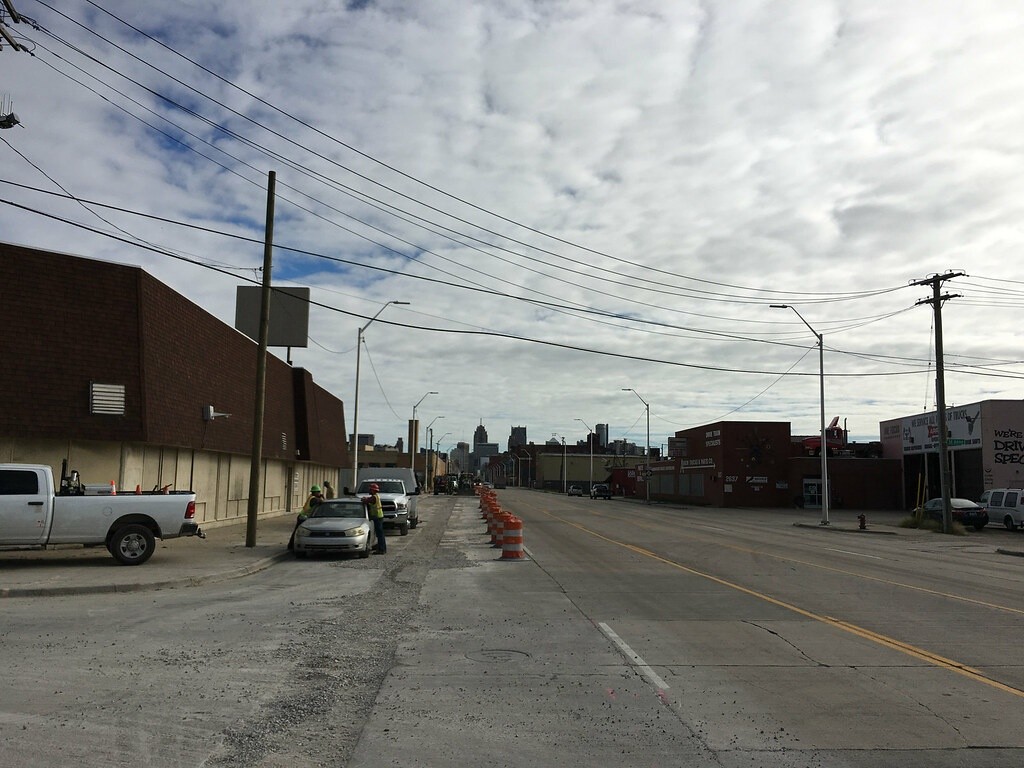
[857,512,867,530]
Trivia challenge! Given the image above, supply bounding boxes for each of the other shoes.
[373,551,385,555]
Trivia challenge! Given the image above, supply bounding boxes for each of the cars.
[568,485,583,497]
[910,497,990,529]
[293,497,376,560]
[483,482,492,489]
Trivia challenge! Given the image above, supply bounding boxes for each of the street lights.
[352,300,412,493]
[520,448,531,488]
[435,432,453,477]
[411,391,439,470]
[551,432,567,493]
[769,304,831,525]
[424,415,446,494]
[622,388,650,505]
[574,418,593,490]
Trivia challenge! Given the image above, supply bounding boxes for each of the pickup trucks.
[0,462,206,566]
[343,477,421,536]
[589,484,614,500]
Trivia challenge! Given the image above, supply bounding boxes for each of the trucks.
[974,488,1024,532]
[801,416,881,458]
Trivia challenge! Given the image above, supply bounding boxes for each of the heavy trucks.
[432,472,476,496]
[493,476,507,489]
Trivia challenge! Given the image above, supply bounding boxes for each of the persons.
[444,480,449,495]
[287,485,325,552]
[324,481,334,508]
[361,483,387,556]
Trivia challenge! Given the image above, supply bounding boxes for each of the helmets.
[368,484,380,491]
[310,485,321,491]
[323,481,329,486]
